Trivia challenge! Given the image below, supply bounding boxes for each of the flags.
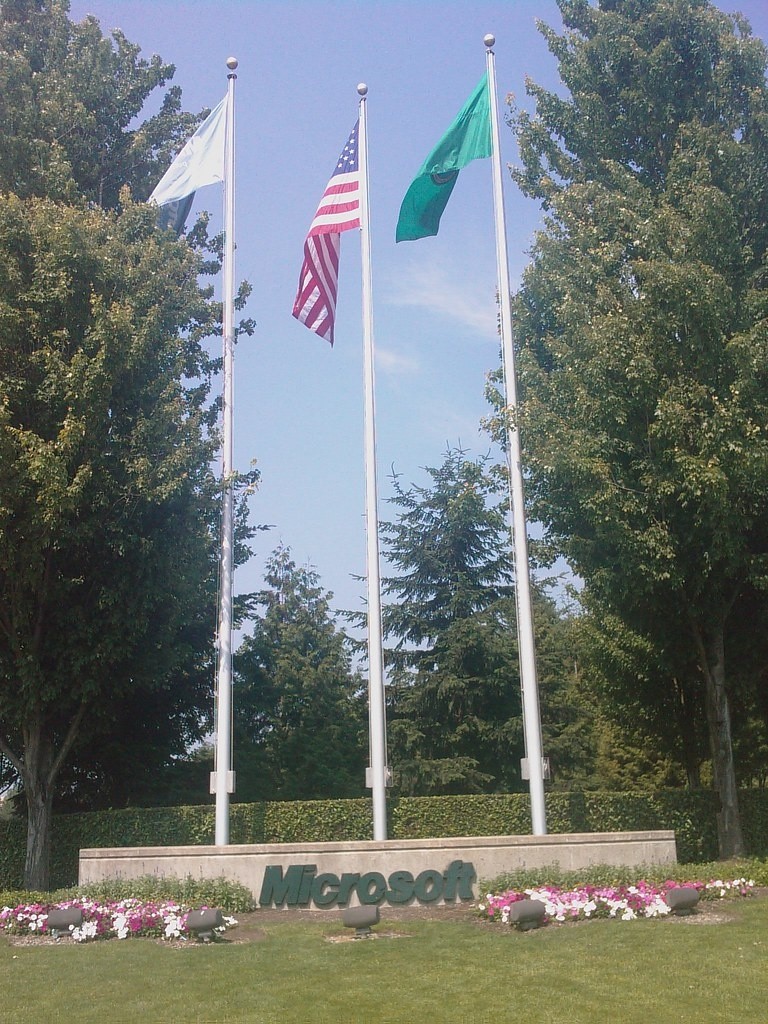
[145,94,228,242]
[395,71,493,244]
[292,118,361,348]
